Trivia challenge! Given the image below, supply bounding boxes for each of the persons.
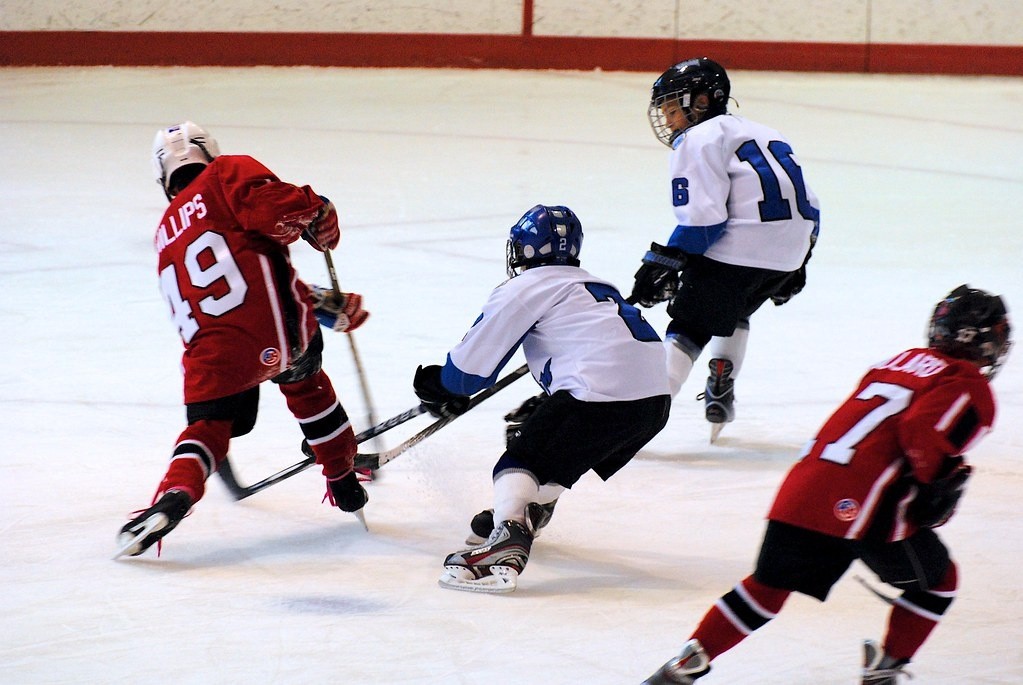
[633,56,820,444]
[108,120,370,562]
[413,203,671,593]
[645,285,1010,685]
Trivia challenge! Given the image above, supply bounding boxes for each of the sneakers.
[464,499,559,546]
[859,640,913,685]
[440,519,534,594]
[640,638,710,685]
[112,489,193,560]
[696,358,735,444]
[327,470,371,533]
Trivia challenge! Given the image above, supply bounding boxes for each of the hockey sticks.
[214,402,430,503]
[315,206,386,455]
[298,282,667,476]
[853,575,898,608]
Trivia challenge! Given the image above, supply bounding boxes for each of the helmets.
[647,57,731,146]
[506,204,583,278]
[152,122,220,202]
[925,284,1010,366]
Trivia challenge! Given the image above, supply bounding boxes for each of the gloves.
[309,283,368,333]
[301,196,340,251]
[902,453,971,531]
[771,235,818,305]
[413,364,472,420]
[630,243,686,308]
